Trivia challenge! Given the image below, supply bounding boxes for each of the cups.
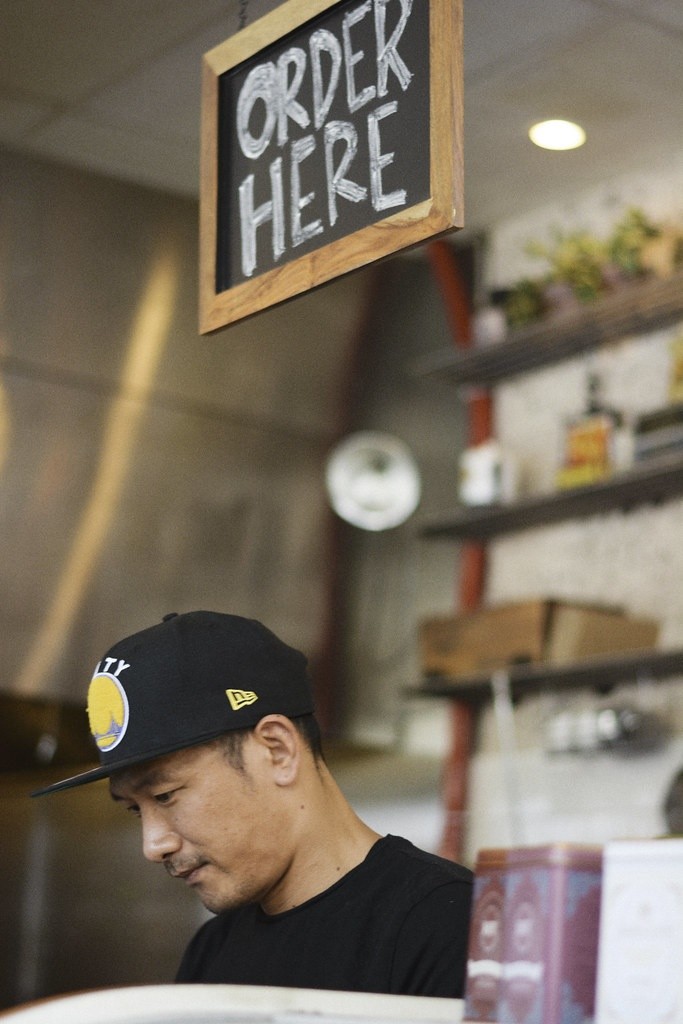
[455,440,519,507]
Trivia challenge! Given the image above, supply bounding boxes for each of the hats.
[29,611,315,798]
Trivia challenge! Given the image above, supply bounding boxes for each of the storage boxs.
[417,595,662,689]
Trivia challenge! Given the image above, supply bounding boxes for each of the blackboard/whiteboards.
[197,1,464,335]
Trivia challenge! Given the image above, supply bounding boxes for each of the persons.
[30,609,475,999]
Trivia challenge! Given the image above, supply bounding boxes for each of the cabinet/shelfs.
[399,267,683,704]
